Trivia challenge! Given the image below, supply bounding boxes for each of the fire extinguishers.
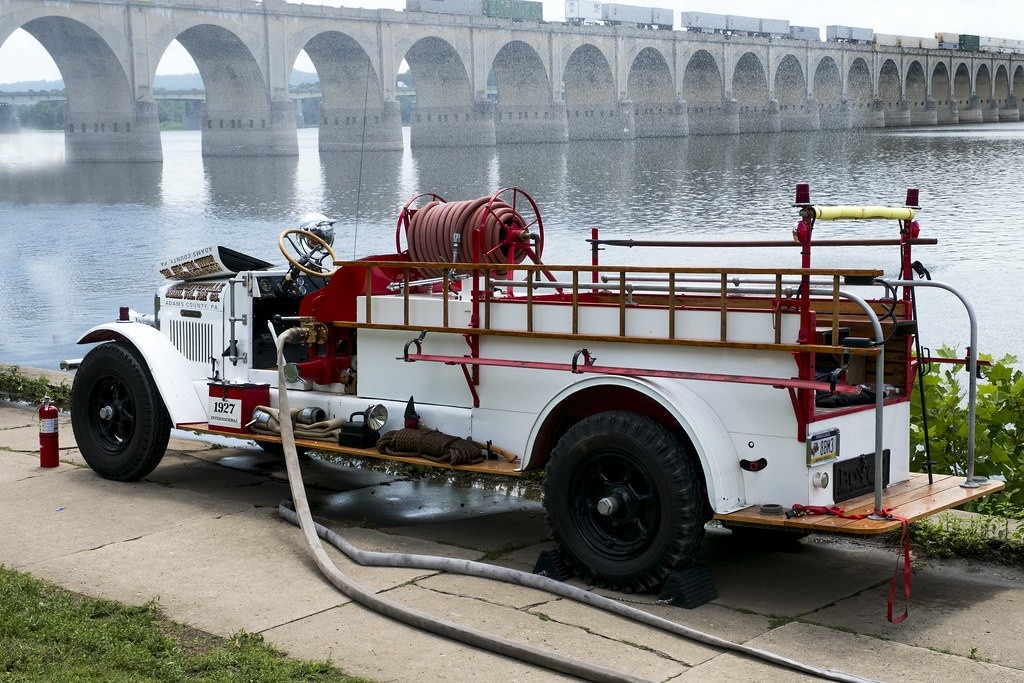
[38,396,60,468]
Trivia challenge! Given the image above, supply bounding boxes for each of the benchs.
[298,254,462,365]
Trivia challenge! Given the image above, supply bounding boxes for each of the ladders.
[332,260,885,356]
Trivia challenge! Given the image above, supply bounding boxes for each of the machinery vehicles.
[55,186,1008,598]
